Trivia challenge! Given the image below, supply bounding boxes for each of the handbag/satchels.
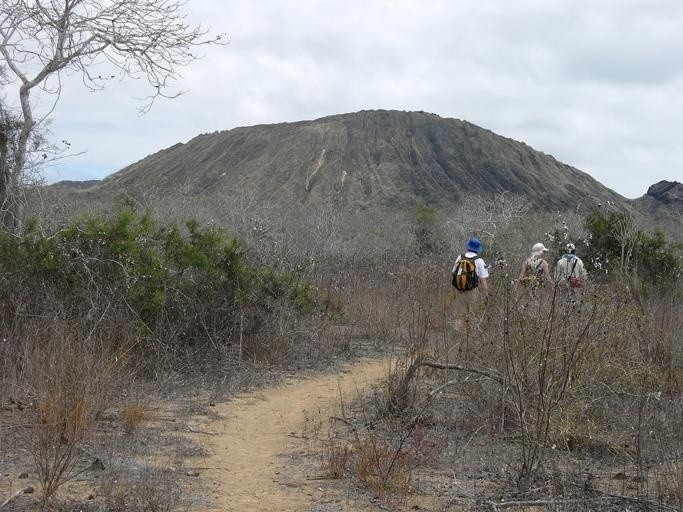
[569,277,586,287]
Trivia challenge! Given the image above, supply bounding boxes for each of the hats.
[565,244,575,251]
[464,239,483,252]
[531,243,549,256]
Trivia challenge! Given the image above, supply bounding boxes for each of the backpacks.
[452,253,481,291]
[522,258,545,290]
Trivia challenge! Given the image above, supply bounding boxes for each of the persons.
[515,243,555,288]
[451,239,489,312]
[554,244,587,288]
